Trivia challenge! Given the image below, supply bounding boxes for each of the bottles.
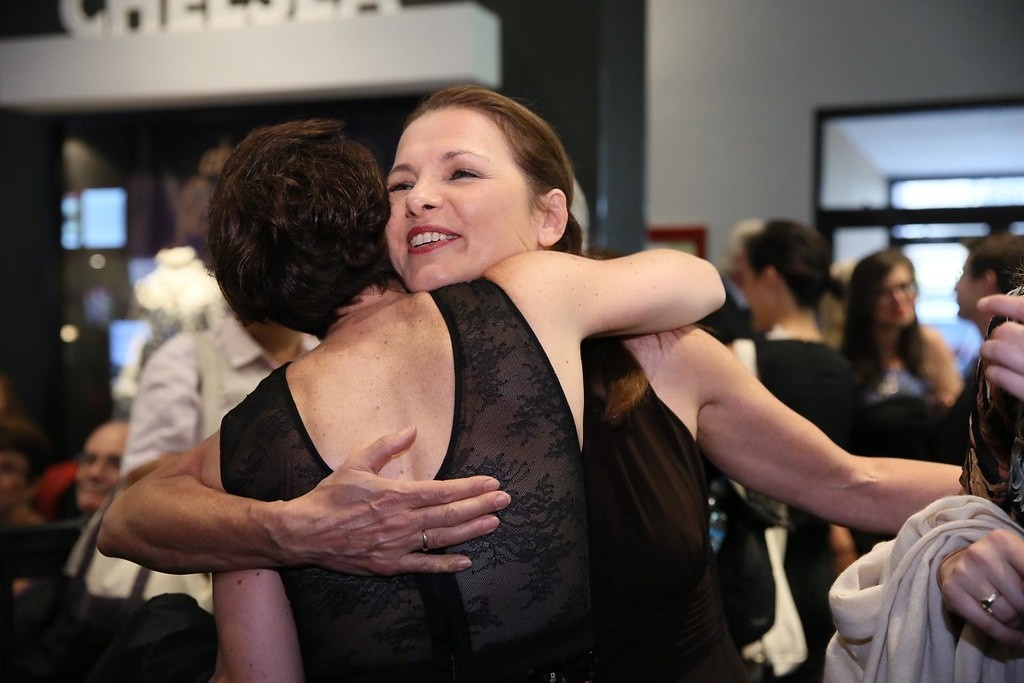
[875,361,909,402]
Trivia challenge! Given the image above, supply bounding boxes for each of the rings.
[422,529,428,551]
[981,592,1000,616]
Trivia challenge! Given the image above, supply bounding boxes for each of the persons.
[0,316,320,608]
[199,119,727,683]
[722,219,1023,683]
[98,89,1023,683]
